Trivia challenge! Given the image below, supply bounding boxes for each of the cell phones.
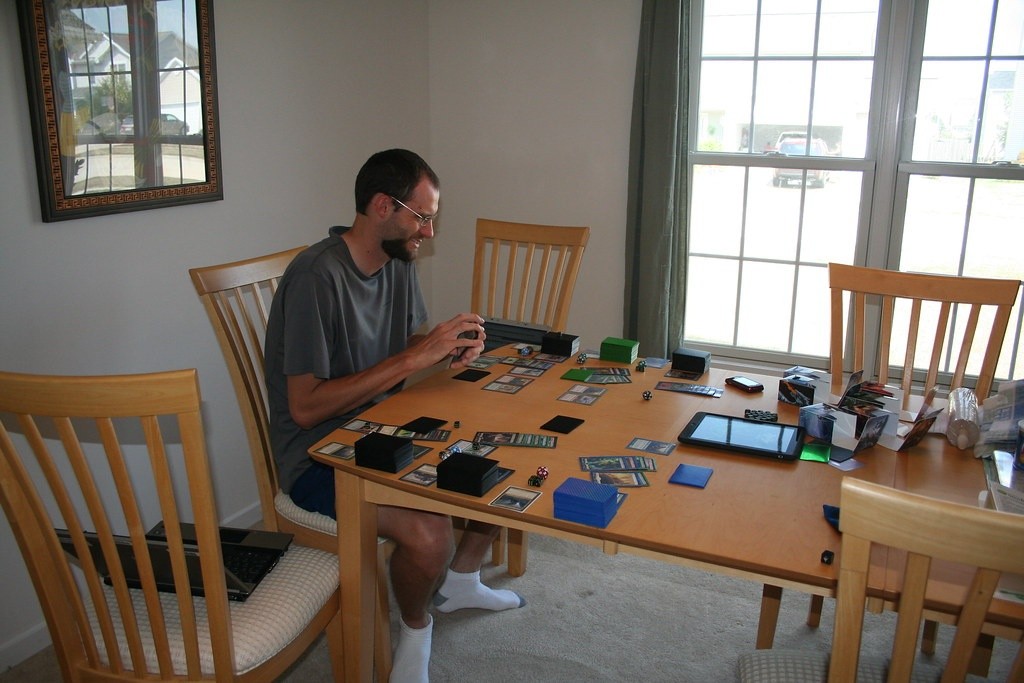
[725,375,764,392]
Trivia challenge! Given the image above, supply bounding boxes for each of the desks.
[308,342,1024,682]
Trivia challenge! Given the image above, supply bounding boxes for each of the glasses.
[392,196,438,224]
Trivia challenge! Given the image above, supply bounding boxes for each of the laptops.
[448,316,551,369]
[54,521,295,602]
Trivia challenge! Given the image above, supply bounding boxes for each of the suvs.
[773,137,830,188]
[120,114,189,135]
[775,131,813,154]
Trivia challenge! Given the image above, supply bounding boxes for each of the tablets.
[678,411,806,460]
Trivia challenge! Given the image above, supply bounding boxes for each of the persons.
[264,149,528,683]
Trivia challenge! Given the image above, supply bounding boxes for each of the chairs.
[736,476,1024,682]
[189,244,469,681]
[0,368,346,682]
[470,217,589,576]
[807,262,1022,657]
